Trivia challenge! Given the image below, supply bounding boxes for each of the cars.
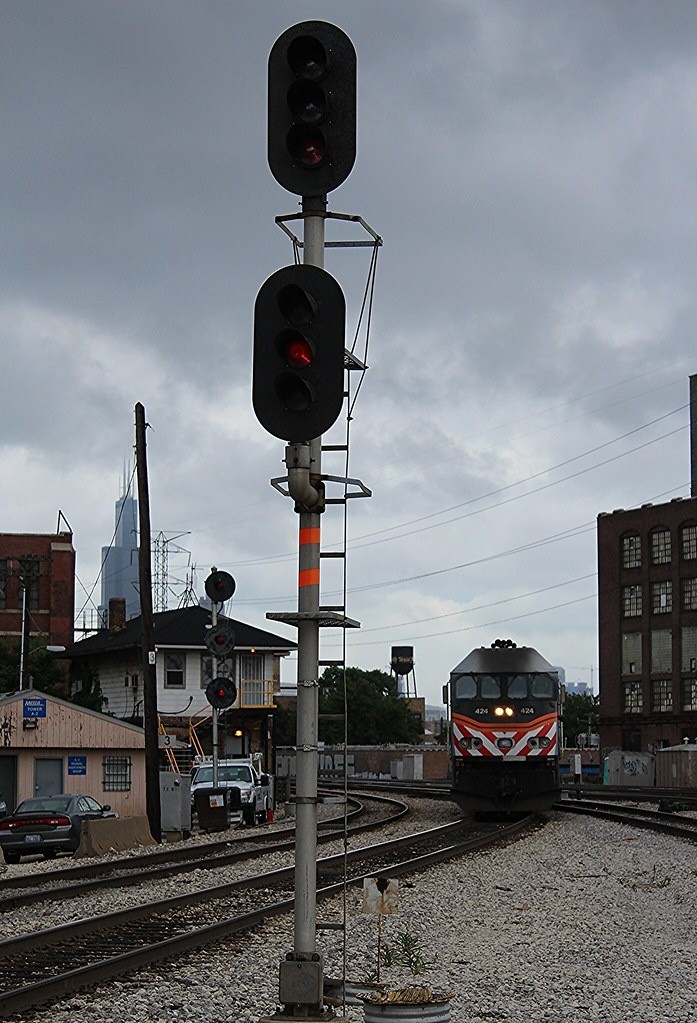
[0,793,120,862]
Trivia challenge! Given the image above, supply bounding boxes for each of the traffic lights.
[204,571,237,602]
[205,624,235,656]
[268,18,357,197]
[250,262,346,445]
[205,678,237,709]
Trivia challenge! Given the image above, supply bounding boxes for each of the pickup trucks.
[190,765,270,824]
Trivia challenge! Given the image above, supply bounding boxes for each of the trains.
[442,639,567,819]
